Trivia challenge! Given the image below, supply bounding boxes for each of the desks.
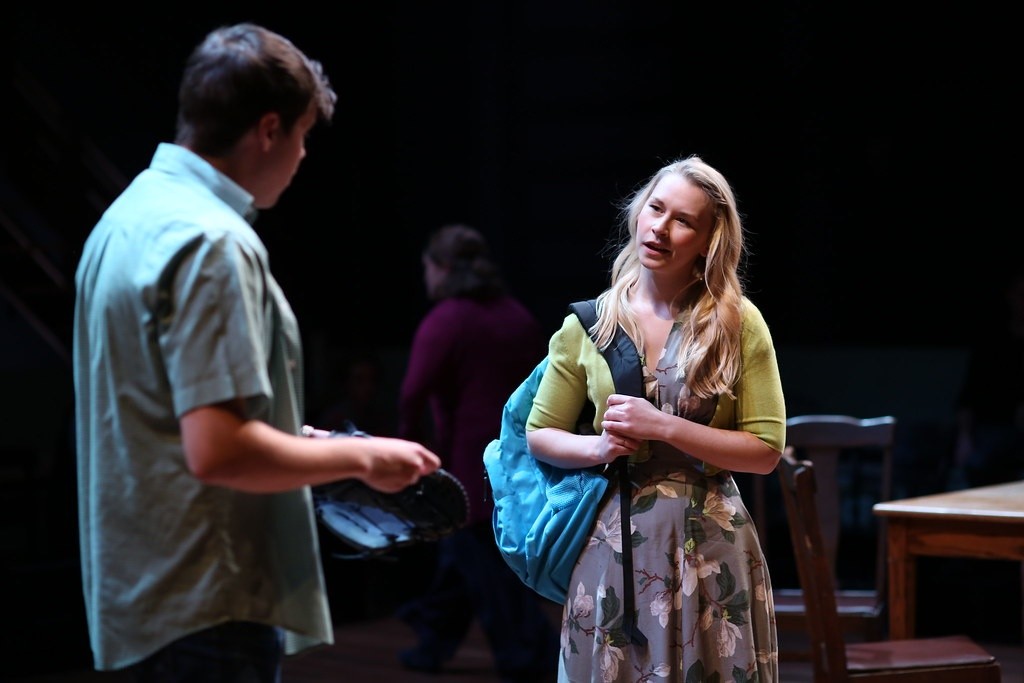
[870,479,1024,641]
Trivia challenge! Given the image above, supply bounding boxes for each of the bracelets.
[301,424,314,439]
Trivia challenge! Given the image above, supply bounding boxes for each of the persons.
[525,157,789,683]
[75,29,442,683]
[400,224,548,670]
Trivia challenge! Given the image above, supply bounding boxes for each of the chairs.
[774,445,1001,683]
[747,411,904,659]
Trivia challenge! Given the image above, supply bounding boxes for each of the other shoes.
[397,643,445,673]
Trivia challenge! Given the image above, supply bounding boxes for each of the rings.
[623,436,627,447]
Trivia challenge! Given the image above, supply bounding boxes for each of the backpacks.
[482,300,648,610]
